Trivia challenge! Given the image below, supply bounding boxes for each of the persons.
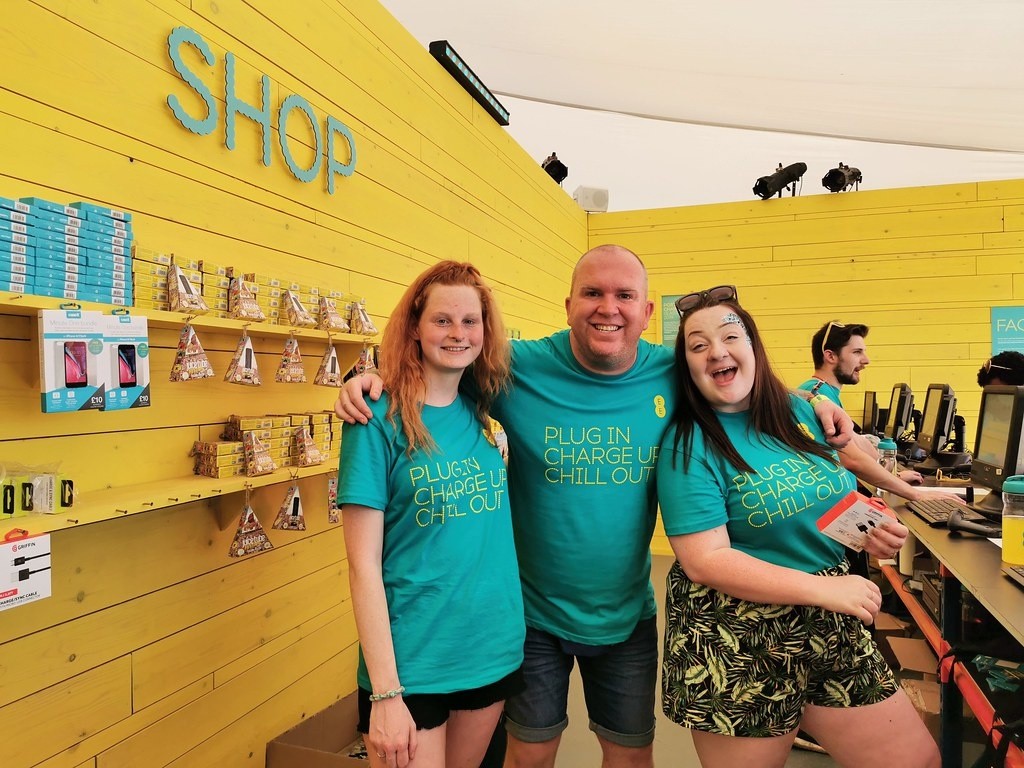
[334,242,857,768]
[657,300,967,768]
[793,318,925,484]
[340,259,527,768]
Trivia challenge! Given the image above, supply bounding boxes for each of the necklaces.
[812,375,826,382]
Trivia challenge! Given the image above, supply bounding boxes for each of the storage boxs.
[37,309,105,413]
[0,194,379,335]
[312,347,343,388]
[100,314,150,410]
[874,612,987,747]
[168,323,214,382]
[274,340,307,383]
[191,411,344,561]
[224,334,261,387]
[353,348,376,375]
[0,533,51,613]
[815,491,897,552]
[264,689,370,768]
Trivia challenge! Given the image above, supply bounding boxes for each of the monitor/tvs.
[913,384,972,474]
[885,382,922,459]
[863,391,879,434]
[969,385,1024,521]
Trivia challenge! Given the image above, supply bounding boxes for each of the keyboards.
[905,498,987,527]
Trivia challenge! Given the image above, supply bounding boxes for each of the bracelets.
[810,394,830,408]
[806,381,824,403]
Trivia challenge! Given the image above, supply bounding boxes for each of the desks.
[863,455,1024,768]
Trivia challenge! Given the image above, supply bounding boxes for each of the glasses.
[821,321,845,355]
[674,284,740,320]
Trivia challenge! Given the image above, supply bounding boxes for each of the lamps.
[822,162,863,194]
[429,41,510,126]
[753,162,808,200]
[541,152,568,185]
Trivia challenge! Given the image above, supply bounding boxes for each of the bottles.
[1001,474,1024,565]
[876,438,897,493]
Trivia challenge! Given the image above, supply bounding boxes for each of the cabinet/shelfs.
[0,289,380,541]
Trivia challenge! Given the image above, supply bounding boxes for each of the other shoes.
[792,724,830,757]
